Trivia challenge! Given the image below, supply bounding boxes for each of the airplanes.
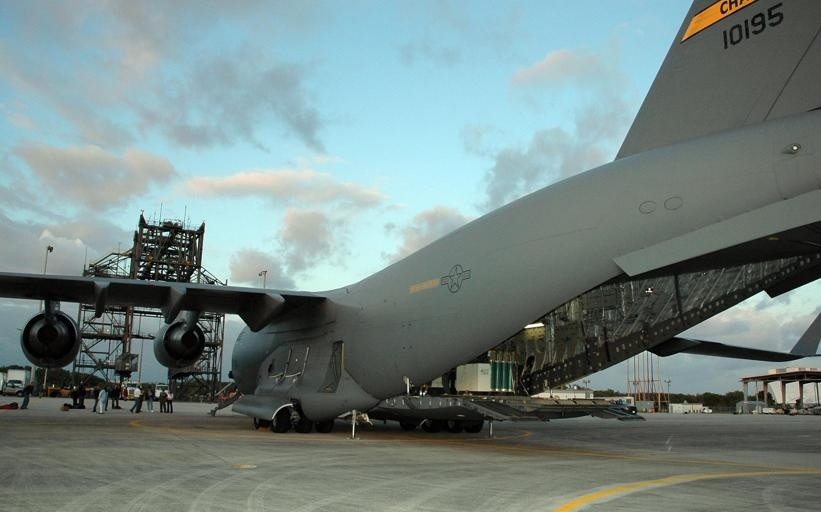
[0,0,820,437]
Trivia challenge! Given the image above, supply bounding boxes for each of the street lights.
[30,244,53,388]
[582,379,590,400]
[663,379,671,404]
[258,270,266,288]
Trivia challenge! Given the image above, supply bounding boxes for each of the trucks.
[142,383,174,402]
[5,369,31,387]
[119,379,140,401]
[0,372,23,397]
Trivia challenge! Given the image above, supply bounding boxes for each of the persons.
[71,384,174,415]
[21,381,36,409]
